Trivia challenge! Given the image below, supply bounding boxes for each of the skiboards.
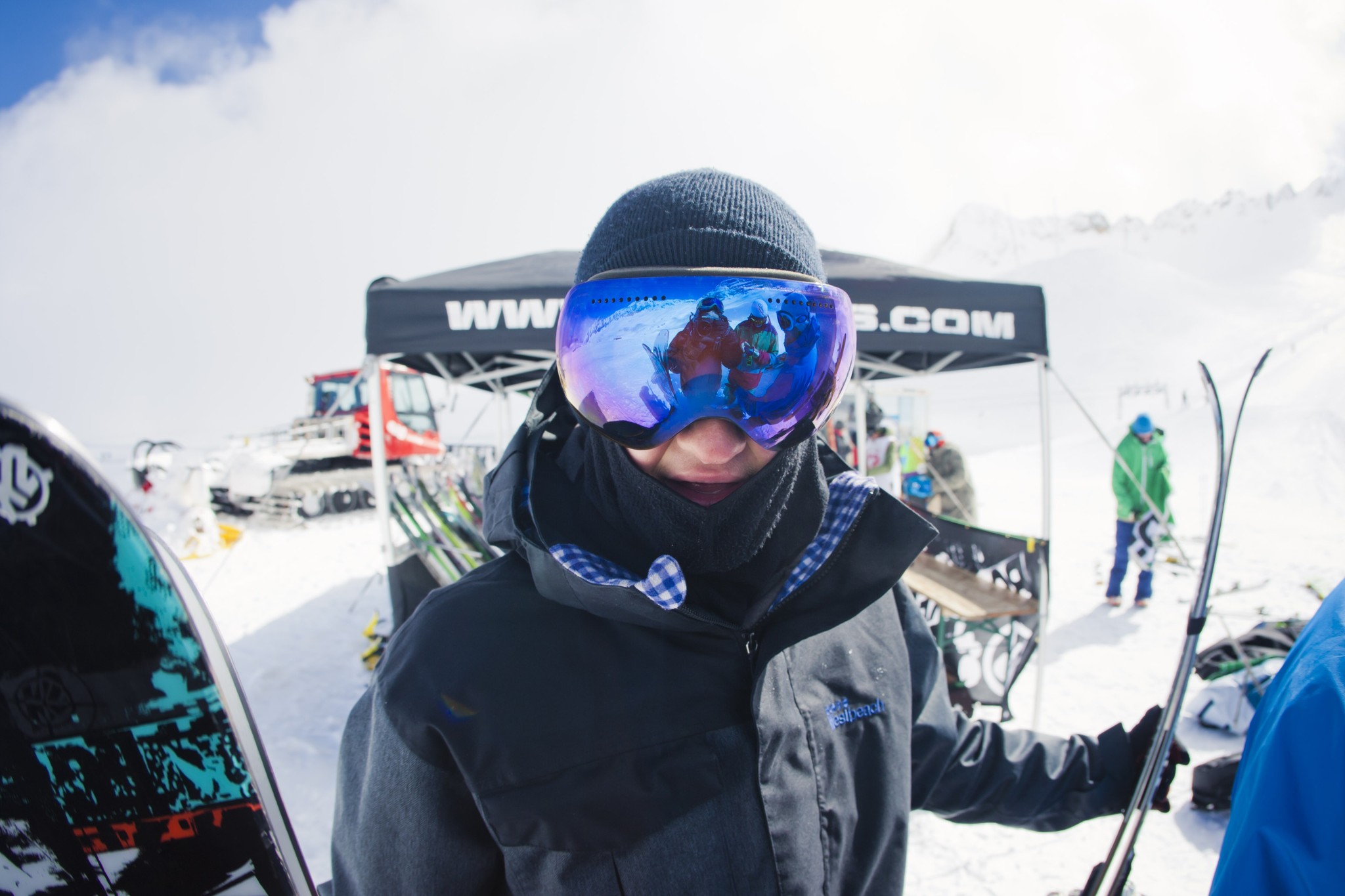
[642,329,676,412]
[387,466,506,587]
[1078,347,1276,895]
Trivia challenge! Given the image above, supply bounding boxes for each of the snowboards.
[0,399,316,896]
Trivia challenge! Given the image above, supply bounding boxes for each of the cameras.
[696,316,723,339]
[744,346,754,366]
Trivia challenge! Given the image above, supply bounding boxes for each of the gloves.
[1130,705,1190,813]
[715,314,728,337]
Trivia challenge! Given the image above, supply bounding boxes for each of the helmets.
[782,292,811,326]
[751,299,767,318]
[695,298,723,317]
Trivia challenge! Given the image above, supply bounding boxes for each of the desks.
[890,550,1041,720]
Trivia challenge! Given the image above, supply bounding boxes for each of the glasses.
[925,436,938,448]
[557,275,856,449]
[776,311,794,331]
[699,298,724,313]
[751,317,767,325]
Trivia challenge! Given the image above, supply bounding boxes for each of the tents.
[366,248,1054,731]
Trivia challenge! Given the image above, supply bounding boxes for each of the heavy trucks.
[127,361,449,520]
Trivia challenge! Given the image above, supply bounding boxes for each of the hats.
[576,168,827,286]
[927,430,945,446]
[1134,415,1153,436]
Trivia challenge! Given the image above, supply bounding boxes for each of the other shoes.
[1137,597,1151,608]
[1108,596,1122,607]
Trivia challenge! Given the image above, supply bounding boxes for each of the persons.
[866,402,902,499]
[332,167,1191,896]
[727,299,779,393]
[1207,577,1345,896]
[835,420,851,460]
[923,430,978,524]
[724,292,820,431]
[668,298,743,407]
[1105,414,1171,607]
